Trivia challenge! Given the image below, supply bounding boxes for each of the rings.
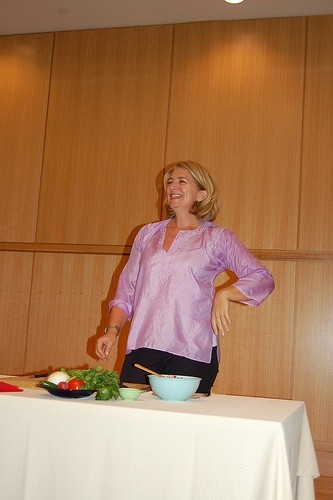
[220,315,224,318]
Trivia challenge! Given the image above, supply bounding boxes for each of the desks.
[0,375,320,500]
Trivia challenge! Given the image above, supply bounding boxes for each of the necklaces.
[172,217,196,226]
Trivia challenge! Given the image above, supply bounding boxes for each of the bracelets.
[104,324,120,337]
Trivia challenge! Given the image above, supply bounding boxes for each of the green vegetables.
[40,366,124,400]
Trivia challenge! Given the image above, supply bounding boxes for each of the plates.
[36,383,97,399]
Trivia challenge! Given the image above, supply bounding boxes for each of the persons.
[95,160,275,397]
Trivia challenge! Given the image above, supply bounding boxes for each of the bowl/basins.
[148,374,202,401]
[118,388,143,401]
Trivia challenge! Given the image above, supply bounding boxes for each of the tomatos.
[68,379,84,391]
[57,382,68,390]
